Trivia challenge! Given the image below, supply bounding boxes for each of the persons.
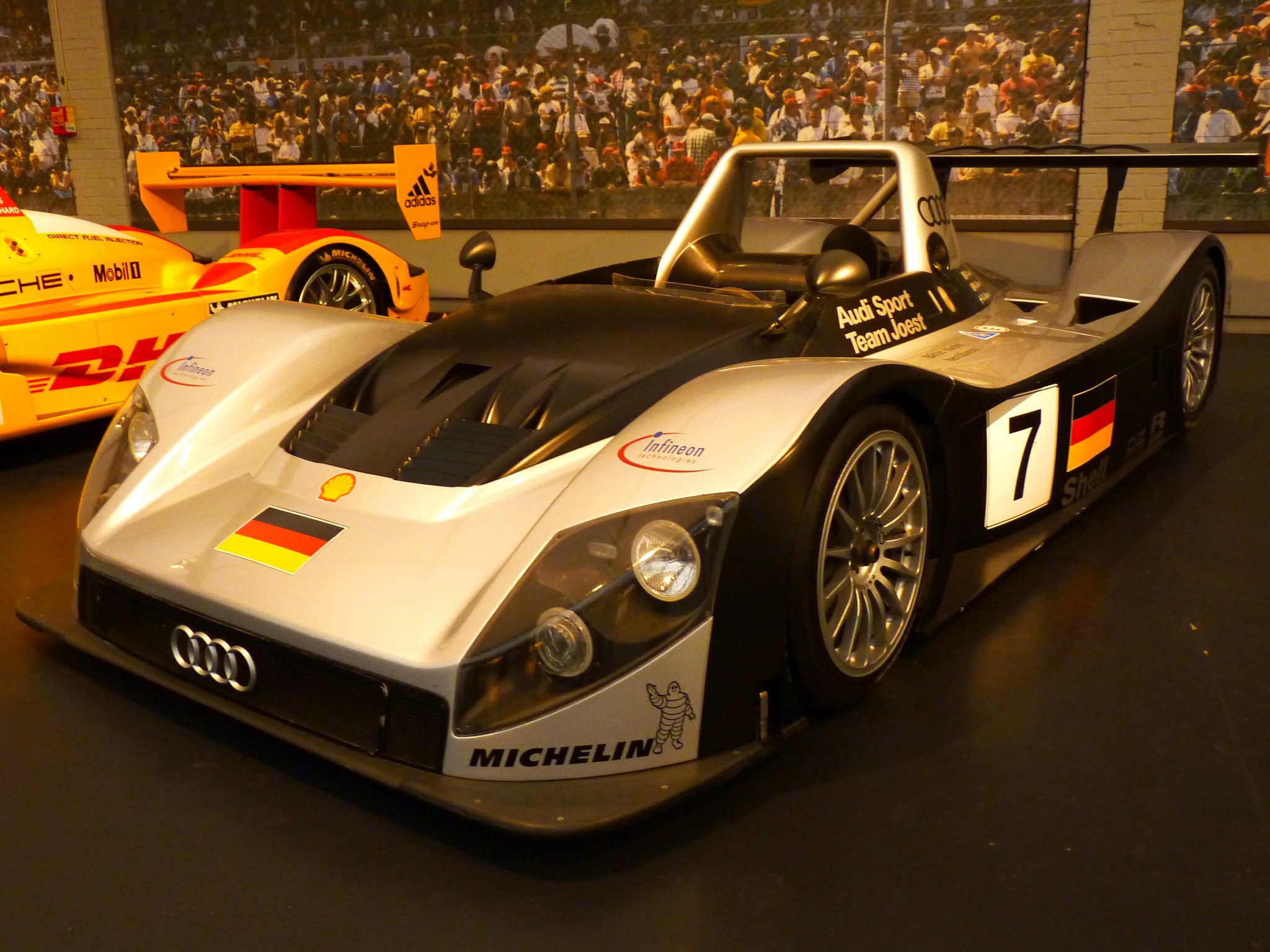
[105,0,1090,218]
[1158,2,1270,224]
[2,0,77,208]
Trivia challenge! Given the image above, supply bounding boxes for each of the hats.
[3,0,1270,187]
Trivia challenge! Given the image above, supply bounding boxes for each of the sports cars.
[1,144,440,435]
[15,140,1262,836]
